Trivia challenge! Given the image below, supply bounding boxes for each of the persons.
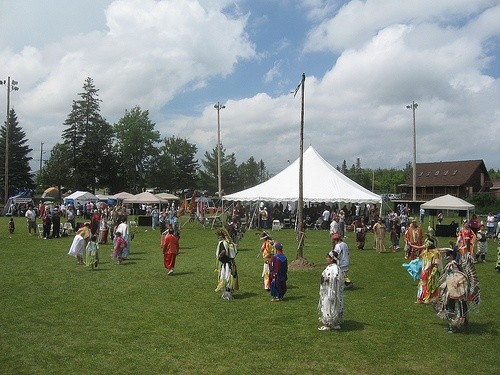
[113,215,130,260]
[214,201,380,299]
[8,218,15,239]
[25,205,37,235]
[420,210,425,224]
[372,217,386,252]
[382,202,500,333]
[142,195,180,275]
[85,235,99,269]
[67,223,90,264]
[317,250,344,330]
[332,233,353,286]
[11,200,129,243]
[438,211,443,225]
[114,232,127,264]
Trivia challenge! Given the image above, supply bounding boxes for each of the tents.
[420,194,475,223]
[114,192,134,209]
[95,194,117,202]
[221,145,383,228]
[63,191,98,207]
[154,193,180,212]
[123,192,169,212]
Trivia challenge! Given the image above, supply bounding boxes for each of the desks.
[434,224,459,238]
[136,215,159,227]
[205,215,222,228]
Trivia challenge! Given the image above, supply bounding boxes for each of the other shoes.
[396,246,401,250]
[333,325,341,329]
[167,269,173,275]
[318,326,330,331]
[482,260,486,263]
[271,297,280,301]
[78,261,86,265]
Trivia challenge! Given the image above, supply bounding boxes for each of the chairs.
[37,222,74,239]
[271,219,281,232]
[284,218,292,229]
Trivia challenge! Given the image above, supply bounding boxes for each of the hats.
[275,243,283,250]
[332,234,340,238]
[114,232,122,236]
